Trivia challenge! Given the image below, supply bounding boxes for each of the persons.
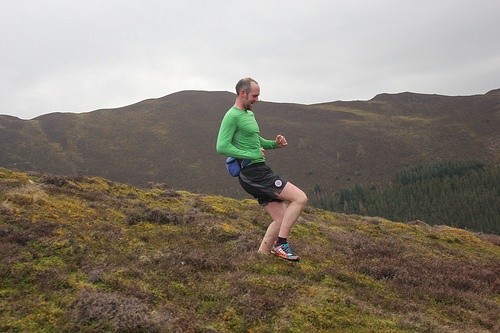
[216,77,307,261]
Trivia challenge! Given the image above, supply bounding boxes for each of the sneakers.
[270,239,300,262]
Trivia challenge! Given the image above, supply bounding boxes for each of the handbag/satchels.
[225,156,249,176]
